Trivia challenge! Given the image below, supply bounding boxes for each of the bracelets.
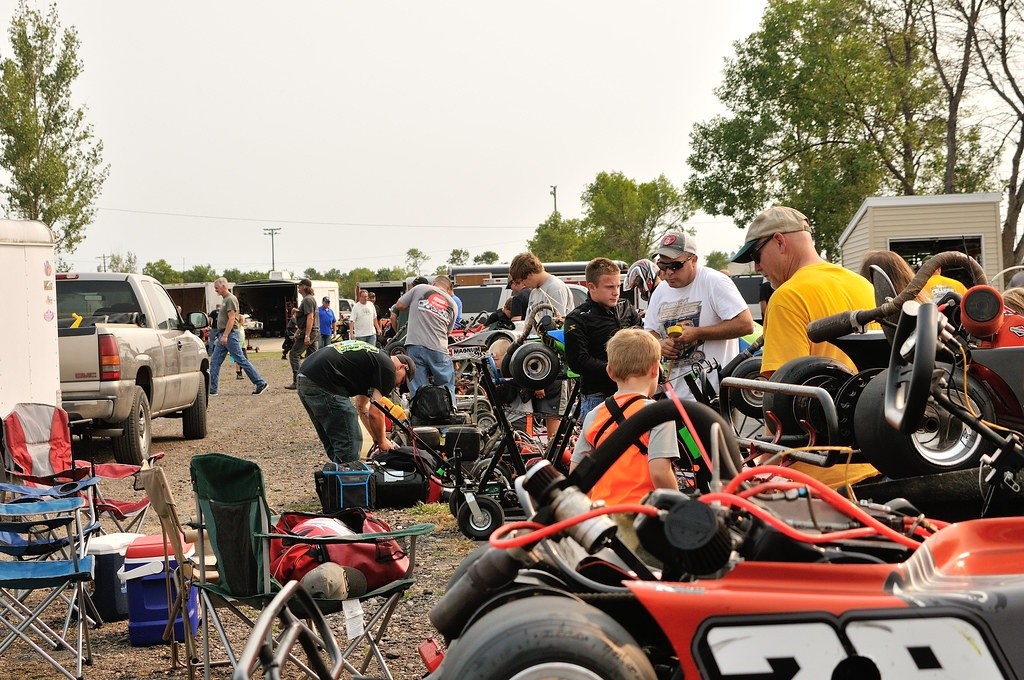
[223,333,228,337]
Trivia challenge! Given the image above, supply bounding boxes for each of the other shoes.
[251,382,269,396]
[208,392,218,397]
[281,354,287,360]
[284,383,296,390]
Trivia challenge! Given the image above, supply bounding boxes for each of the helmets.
[622,259,662,302]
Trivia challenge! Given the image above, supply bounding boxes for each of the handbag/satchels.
[409,383,458,425]
[268,507,415,600]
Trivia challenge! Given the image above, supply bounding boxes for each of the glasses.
[324,301,330,304]
[656,255,693,271]
[749,234,774,264]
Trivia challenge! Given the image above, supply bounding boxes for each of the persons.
[176,305,182,313]
[731,207,884,492]
[564,258,645,429]
[569,326,680,568]
[349,289,382,347]
[482,251,576,441]
[281,280,336,389]
[916,256,968,304]
[623,258,660,316]
[642,232,754,403]
[297,339,416,464]
[859,251,931,325]
[391,275,462,437]
[209,277,269,397]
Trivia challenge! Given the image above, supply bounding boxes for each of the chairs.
[2,402,166,536]
[185,453,434,680]
[142,455,314,680]
[0,477,105,649]
[0,500,96,680]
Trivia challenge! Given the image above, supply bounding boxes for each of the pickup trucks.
[338,297,357,320]
[54,269,213,470]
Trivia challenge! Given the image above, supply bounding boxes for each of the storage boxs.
[75,530,145,622]
[125,532,200,647]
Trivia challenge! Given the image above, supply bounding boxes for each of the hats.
[506,274,516,290]
[730,205,812,266]
[397,353,416,393]
[651,231,697,257]
[291,307,299,314]
[322,295,331,302]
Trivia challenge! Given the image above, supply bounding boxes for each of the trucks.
[442,259,661,353]
